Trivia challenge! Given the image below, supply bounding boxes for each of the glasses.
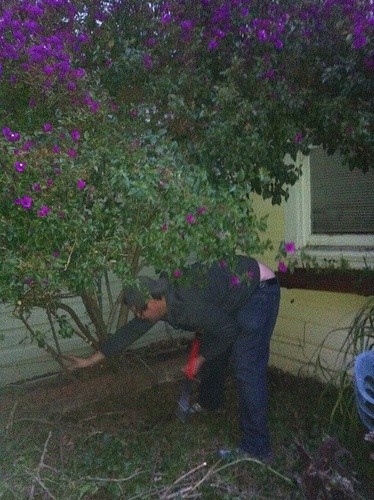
[136,309,145,320]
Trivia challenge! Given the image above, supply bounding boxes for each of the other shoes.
[189,402,227,415]
[219,447,271,458]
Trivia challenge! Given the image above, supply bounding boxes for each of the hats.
[121,275,173,303]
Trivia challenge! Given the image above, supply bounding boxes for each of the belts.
[259,278,279,288]
